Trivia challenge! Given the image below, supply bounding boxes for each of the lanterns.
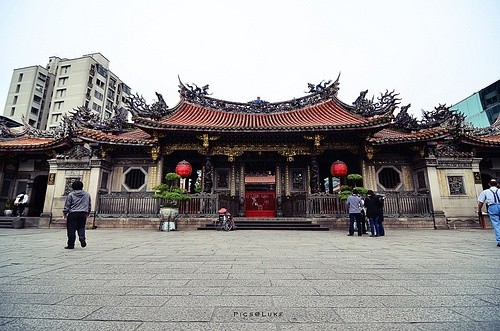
[175,160,192,179]
[331,160,348,179]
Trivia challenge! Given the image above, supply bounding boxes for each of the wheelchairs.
[215,213,234,231]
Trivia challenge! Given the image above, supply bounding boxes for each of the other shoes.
[347,233,385,237]
[79,236,86,247]
[64,245,74,249]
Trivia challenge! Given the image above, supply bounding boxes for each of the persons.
[9,190,29,217]
[345,188,384,236]
[63,181,91,249]
[478,179,500,246]
